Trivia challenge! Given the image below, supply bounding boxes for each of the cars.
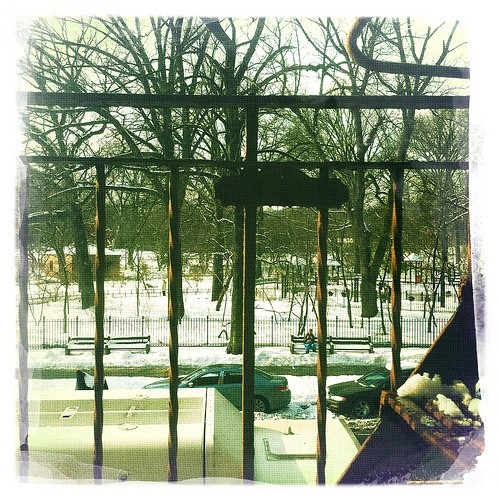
[326,362,416,419]
[142,365,291,413]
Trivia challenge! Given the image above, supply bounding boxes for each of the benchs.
[65,335,150,356]
[290,335,373,355]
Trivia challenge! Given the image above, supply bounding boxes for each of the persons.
[303,329,317,353]
[162,280,166,296]
